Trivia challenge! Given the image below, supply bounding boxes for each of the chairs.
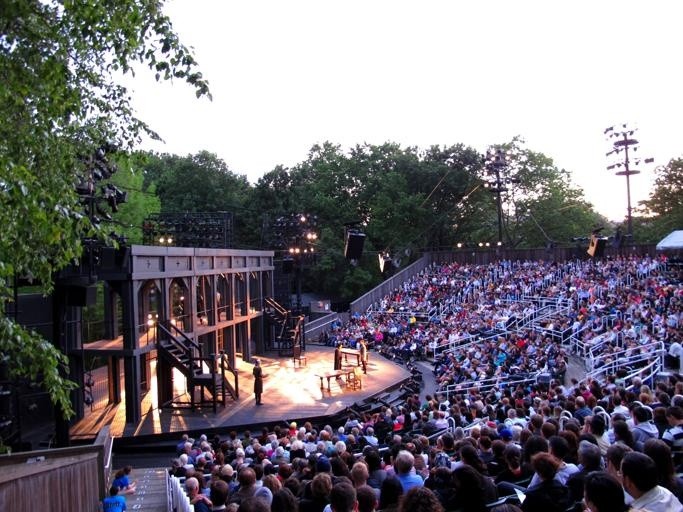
[344,366,361,391]
[292,344,307,368]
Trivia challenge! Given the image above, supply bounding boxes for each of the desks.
[339,348,371,366]
[315,368,355,391]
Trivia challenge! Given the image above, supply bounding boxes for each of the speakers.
[344,232,366,258]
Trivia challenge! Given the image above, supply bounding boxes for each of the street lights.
[605,124,655,243]
[480,149,522,249]
[288,214,318,314]
[53,144,127,447]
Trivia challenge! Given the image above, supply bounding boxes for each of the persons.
[112,465,137,495]
[102,486,127,512]
